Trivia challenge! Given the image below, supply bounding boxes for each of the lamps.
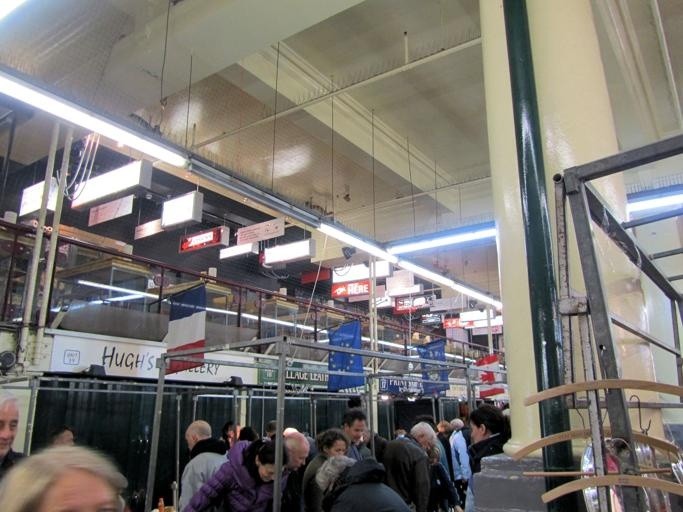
[0,71,191,172]
[313,219,505,315]
[619,183,682,216]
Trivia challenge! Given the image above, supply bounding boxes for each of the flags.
[474,352,505,400]
[325,318,365,391]
[162,284,207,375]
[415,338,449,394]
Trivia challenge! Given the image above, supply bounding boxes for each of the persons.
[0,395,128,511]
[178,401,512,511]
